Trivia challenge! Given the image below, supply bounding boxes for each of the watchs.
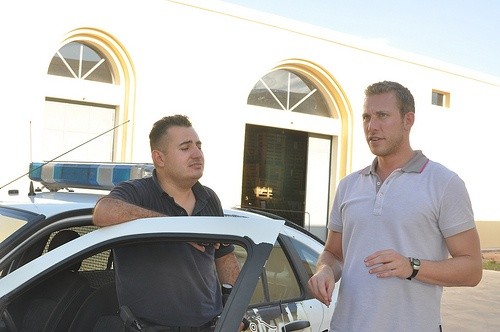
[407,256,420,282]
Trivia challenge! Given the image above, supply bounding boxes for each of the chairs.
[13,230,90,332]
[67,284,122,332]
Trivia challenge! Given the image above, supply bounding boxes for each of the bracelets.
[241,316,251,329]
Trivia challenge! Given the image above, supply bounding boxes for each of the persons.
[91,115,251,332]
[307,81,482,332]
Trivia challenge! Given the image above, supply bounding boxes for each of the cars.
[0,160,342,332]
